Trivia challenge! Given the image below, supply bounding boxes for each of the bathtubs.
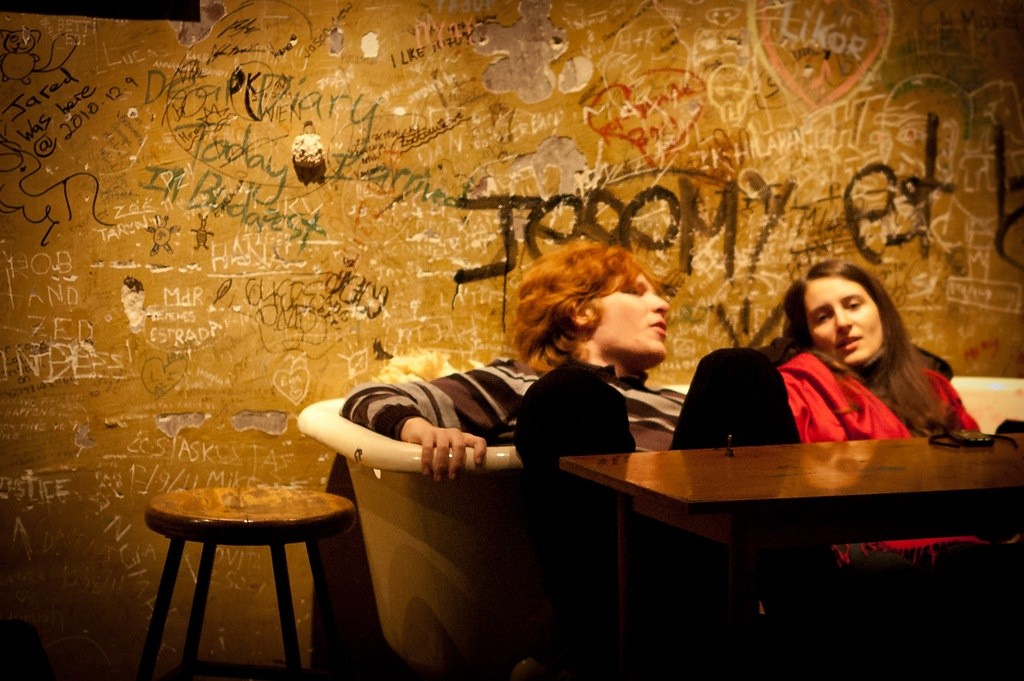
[295,374,1024,675]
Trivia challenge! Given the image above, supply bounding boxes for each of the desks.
[557,433,1024,652]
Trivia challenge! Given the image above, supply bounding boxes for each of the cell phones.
[951,429,996,447]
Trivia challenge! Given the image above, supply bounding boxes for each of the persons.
[777,261,1023,681]
[342,242,800,681]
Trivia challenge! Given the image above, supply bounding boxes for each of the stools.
[136,486,357,681]
